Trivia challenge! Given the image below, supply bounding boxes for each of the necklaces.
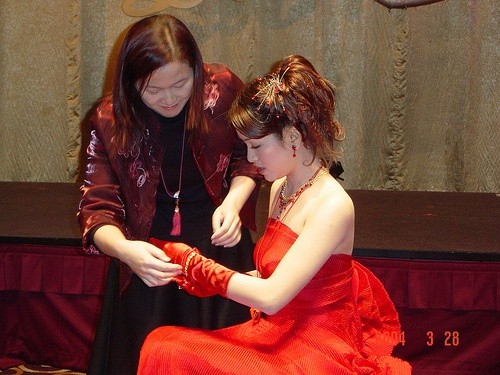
[274,165,328,222]
[151,105,188,235]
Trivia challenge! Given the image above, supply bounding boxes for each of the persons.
[136,55,411,375]
[78,15,263,375]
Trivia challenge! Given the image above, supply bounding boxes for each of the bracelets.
[183,252,197,277]
